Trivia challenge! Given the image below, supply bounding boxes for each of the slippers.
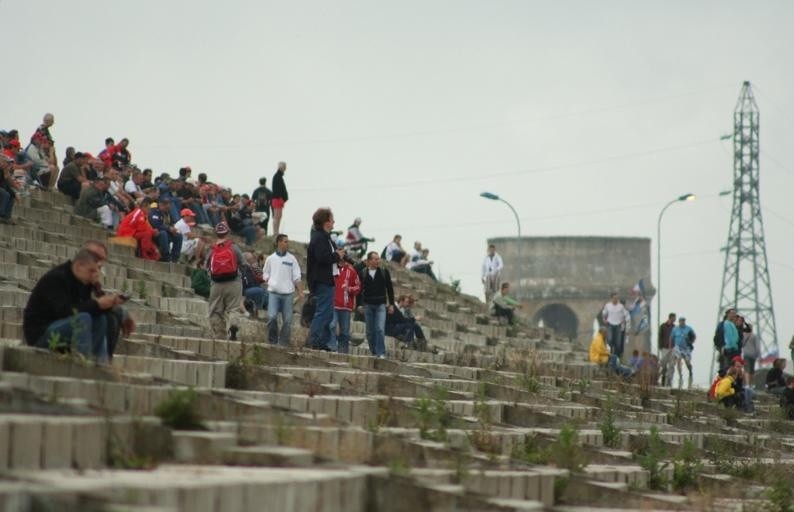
[210,239,237,282]
[714,322,725,346]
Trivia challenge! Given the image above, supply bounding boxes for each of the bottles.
[216,221,229,234]
[181,208,195,217]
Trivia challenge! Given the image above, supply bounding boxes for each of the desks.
[475,189,531,308]
[654,189,698,375]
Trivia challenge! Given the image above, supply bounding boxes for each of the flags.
[758,350,779,368]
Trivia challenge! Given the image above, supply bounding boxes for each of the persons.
[657,313,696,390]
[766,358,793,420]
[22,238,122,369]
[490,283,522,327]
[384,295,432,352]
[714,309,761,418]
[2,113,288,318]
[589,292,644,384]
[380,234,438,282]
[262,233,304,349]
[203,220,248,340]
[304,208,395,358]
[480,244,503,306]
